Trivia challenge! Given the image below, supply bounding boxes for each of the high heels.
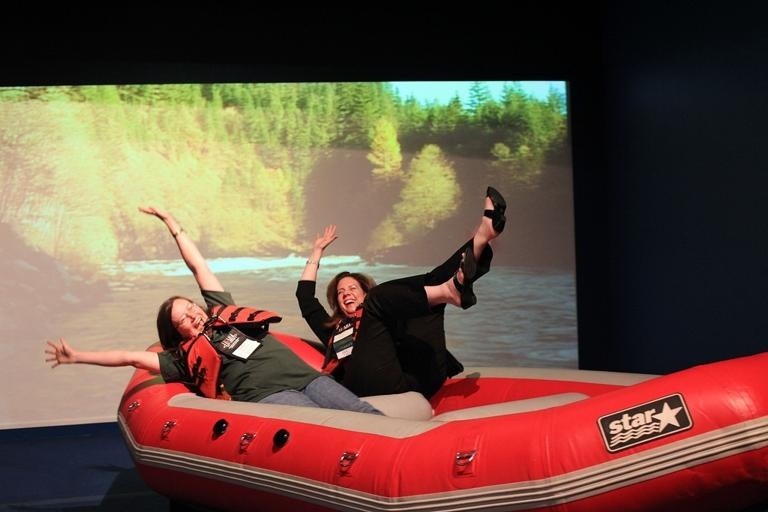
[453,247,477,310]
[484,186,507,233]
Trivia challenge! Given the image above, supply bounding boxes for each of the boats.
[117,330,768,512]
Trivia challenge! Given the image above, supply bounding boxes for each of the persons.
[42,204,382,419]
[295,186,506,400]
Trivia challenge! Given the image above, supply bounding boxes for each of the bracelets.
[171,227,183,238]
[304,259,319,268]
